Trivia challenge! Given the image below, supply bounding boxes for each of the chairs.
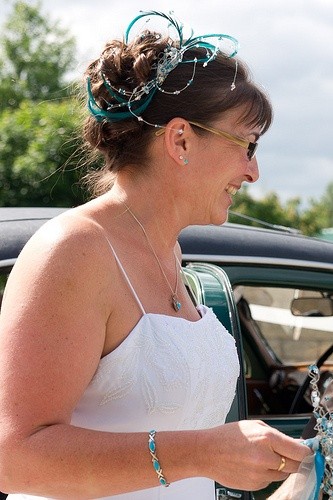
[237,296,326,418]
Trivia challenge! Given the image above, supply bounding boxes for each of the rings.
[278,456,286,472]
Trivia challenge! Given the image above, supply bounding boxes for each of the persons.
[1,28,314,500]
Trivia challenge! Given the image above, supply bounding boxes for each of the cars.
[0,204,332,500]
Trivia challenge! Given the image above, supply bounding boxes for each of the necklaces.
[110,190,181,313]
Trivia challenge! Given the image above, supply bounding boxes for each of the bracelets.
[149,430,171,488]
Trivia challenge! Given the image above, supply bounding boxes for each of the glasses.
[154,120,259,162]
